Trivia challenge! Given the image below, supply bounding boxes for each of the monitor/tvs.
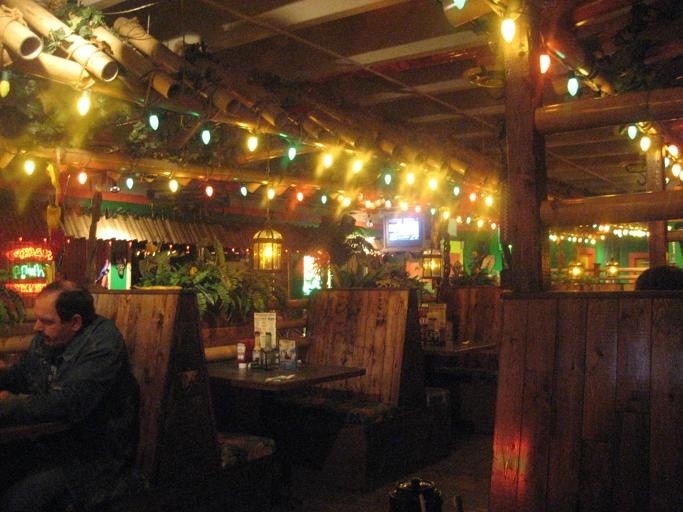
[385,215,422,247]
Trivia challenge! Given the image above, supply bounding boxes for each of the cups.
[236,338,255,368]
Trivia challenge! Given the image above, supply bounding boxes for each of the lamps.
[253,144,283,273]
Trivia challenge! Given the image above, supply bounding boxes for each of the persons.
[634,265,683,289]
[1,280,142,509]
[468,250,482,276]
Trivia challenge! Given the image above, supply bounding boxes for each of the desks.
[0,419,66,444]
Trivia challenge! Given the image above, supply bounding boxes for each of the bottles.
[250,330,275,371]
